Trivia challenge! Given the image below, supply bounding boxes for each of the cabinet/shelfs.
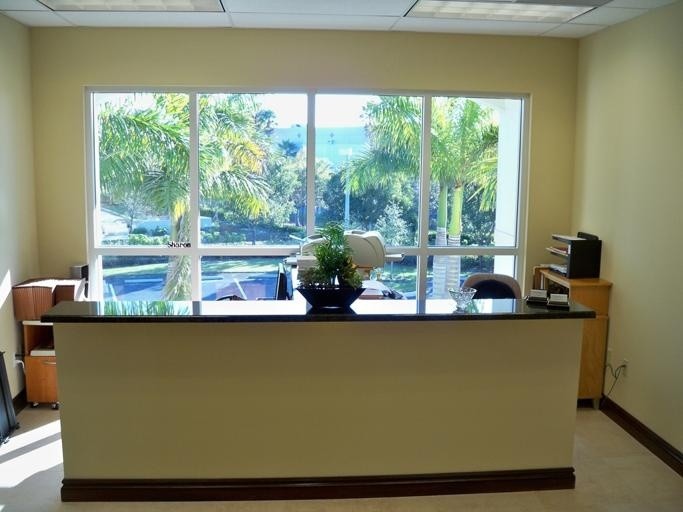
[18,318,59,411]
[533,265,615,412]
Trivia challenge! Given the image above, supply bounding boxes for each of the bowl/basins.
[448,287,477,308]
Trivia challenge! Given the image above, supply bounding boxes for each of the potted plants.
[296,220,367,308]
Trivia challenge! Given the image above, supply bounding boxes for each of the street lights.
[340,147,354,229]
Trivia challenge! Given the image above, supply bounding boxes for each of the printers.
[285,230,386,282]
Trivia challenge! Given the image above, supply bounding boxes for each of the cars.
[357,279,389,298]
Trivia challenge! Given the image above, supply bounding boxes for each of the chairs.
[462,273,521,299]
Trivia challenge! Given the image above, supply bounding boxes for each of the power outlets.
[621,359,628,376]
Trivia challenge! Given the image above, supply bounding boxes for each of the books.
[546,234,586,272]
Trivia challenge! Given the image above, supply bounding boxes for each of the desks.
[288,253,390,300]
